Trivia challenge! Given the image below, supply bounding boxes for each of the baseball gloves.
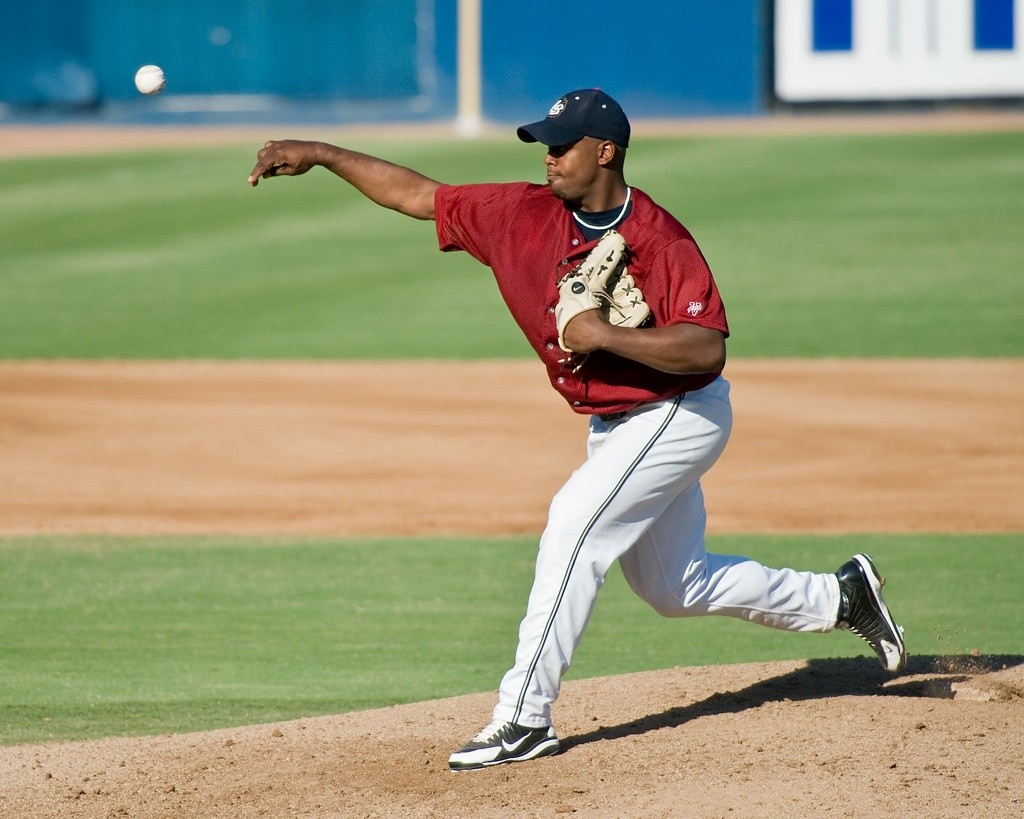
[551,225,651,358]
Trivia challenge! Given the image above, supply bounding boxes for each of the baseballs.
[132,62,166,95]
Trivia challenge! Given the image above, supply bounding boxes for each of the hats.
[516,87,631,149]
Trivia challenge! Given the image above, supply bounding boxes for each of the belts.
[598,411,627,421]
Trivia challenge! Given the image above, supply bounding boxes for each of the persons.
[246,88,909,772]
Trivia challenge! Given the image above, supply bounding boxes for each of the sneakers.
[833,552,910,673]
[447,720,561,772]
